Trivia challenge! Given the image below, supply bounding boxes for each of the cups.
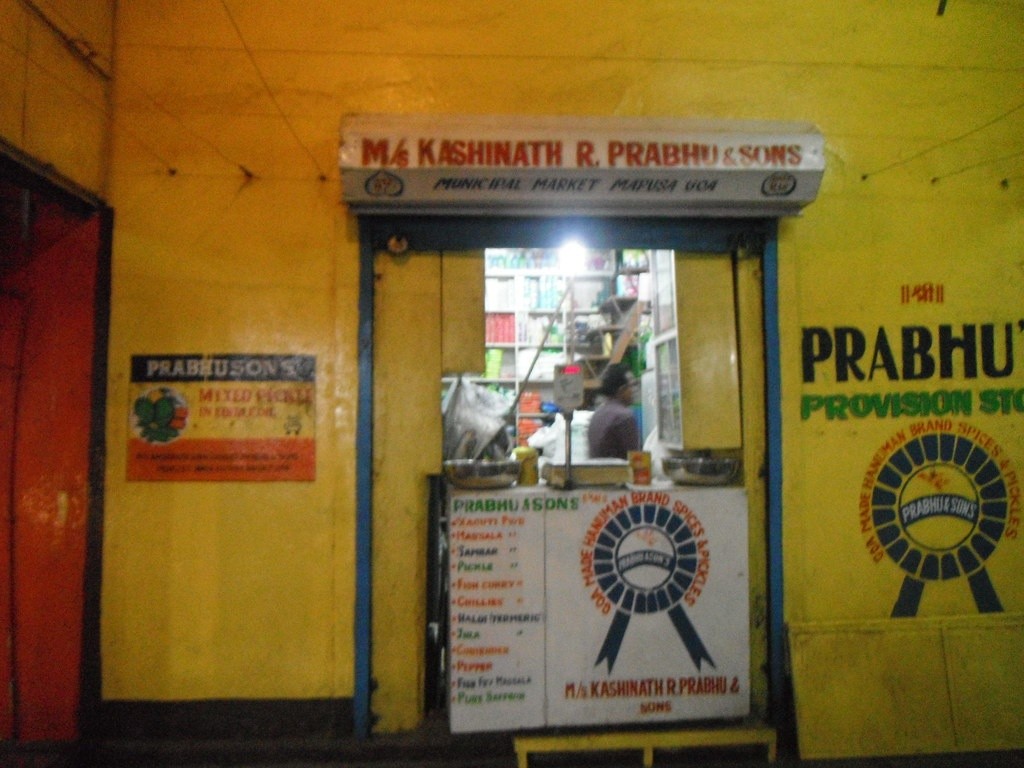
[627,451,651,486]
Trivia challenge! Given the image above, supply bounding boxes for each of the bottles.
[488,246,557,268]
[514,437,538,485]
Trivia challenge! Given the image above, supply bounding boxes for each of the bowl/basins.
[444,458,519,488]
[662,456,740,487]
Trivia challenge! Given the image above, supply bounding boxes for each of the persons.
[588,363,640,459]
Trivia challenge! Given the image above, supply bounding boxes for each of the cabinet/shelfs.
[485,247,681,454]
